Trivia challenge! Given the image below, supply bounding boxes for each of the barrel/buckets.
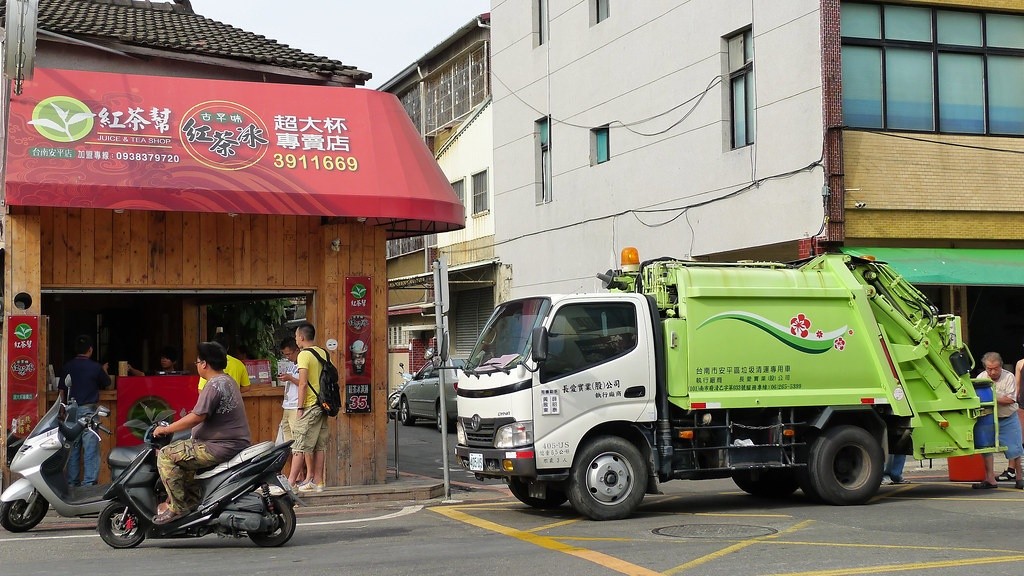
[947,452,988,481]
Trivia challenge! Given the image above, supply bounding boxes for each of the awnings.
[5,67,465,240]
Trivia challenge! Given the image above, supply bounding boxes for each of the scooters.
[97,421,308,549]
[386,362,415,421]
[0,375,191,533]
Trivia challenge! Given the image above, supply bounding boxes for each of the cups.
[349,347,368,374]
[118,361,128,376]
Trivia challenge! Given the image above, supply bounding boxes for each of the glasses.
[283,349,297,356]
[194,361,204,365]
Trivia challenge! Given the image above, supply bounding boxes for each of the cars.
[399,354,471,435]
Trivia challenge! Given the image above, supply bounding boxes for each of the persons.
[881,453,912,485]
[975,352,1024,489]
[58,335,109,488]
[127,332,251,524]
[274,322,333,493]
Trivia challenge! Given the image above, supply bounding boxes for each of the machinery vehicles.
[423,247,1009,524]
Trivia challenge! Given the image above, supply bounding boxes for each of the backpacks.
[304,348,342,417]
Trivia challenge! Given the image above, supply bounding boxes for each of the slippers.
[1014,480,1024,491]
[972,480,998,489]
[152,508,191,525]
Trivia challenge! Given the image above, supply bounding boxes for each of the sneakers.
[289,483,297,495]
[879,475,894,485]
[300,479,312,486]
[299,478,325,492]
[996,469,1016,482]
[894,477,911,484]
